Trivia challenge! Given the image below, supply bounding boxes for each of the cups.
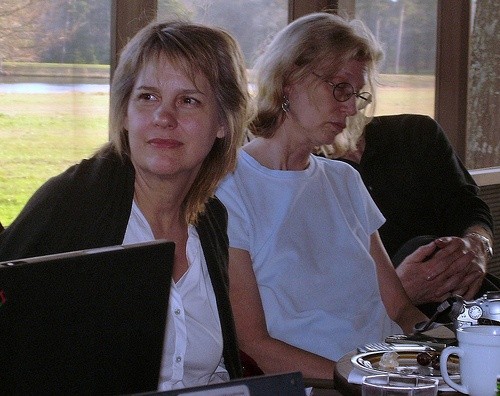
[440,325,500,396]
[360,374,438,396]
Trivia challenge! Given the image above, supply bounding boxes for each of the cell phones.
[384,335,458,348]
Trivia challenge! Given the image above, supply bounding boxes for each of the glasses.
[309,71,373,111]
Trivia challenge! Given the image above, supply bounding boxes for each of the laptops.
[0,241,178,396]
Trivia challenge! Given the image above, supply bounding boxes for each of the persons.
[207,12,457,379]
[0,20,265,393]
[312,111,500,324]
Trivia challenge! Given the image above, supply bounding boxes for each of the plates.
[350,346,461,382]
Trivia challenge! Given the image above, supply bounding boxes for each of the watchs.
[467,232,493,260]
